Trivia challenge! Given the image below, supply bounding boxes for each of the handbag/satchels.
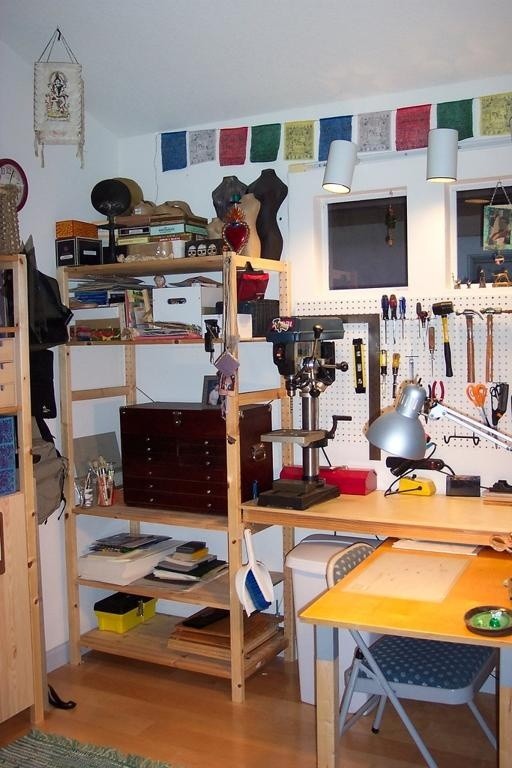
[15,438,66,525]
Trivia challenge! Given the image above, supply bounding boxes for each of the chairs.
[327,543,497,768]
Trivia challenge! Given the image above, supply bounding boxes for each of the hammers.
[480,307,502,383]
[432,301,453,377]
[455,308,484,383]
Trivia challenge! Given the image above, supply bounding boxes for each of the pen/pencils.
[84,455,115,506]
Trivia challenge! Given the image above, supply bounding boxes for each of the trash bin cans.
[285,534,386,716]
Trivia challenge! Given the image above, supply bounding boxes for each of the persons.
[236,193,262,258]
[491,216,510,245]
[205,217,226,240]
[208,383,220,406]
[211,176,247,225]
[245,169,288,261]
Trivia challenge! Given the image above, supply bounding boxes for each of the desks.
[241,491,512,663]
[298,538,511,768]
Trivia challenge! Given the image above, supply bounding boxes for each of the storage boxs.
[55,238,103,268]
[93,592,156,634]
[151,285,278,339]
[55,220,99,239]
[119,402,273,517]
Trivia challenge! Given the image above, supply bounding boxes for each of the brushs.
[245,529,271,611]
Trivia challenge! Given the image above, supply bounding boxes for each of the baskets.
[0,182,22,255]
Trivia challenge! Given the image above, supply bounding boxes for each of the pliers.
[419,381,445,424]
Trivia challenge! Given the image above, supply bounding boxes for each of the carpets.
[0,730,173,768]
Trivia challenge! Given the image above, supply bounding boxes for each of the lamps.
[321,140,360,195]
[426,128,462,183]
[365,384,512,461]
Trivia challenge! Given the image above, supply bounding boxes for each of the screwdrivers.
[392,353,400,399]
[380,350,387,399]
[429,327,435,377]
[416,302,422,338]
[381,294,406,345]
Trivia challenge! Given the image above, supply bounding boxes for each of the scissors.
[465,383,491,428]
[489,385,502,401]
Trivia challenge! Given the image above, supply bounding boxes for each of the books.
[69,274,223,340]
[392,539,484,556]
[78,532,228,593]
[481,489,512,507]
[92,214,209,247]
[166,606,284,662]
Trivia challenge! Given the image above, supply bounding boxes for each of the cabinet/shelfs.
[58,251,296,703]
[0,255,48,726]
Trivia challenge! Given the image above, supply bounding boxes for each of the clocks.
[0,159,28,212]
